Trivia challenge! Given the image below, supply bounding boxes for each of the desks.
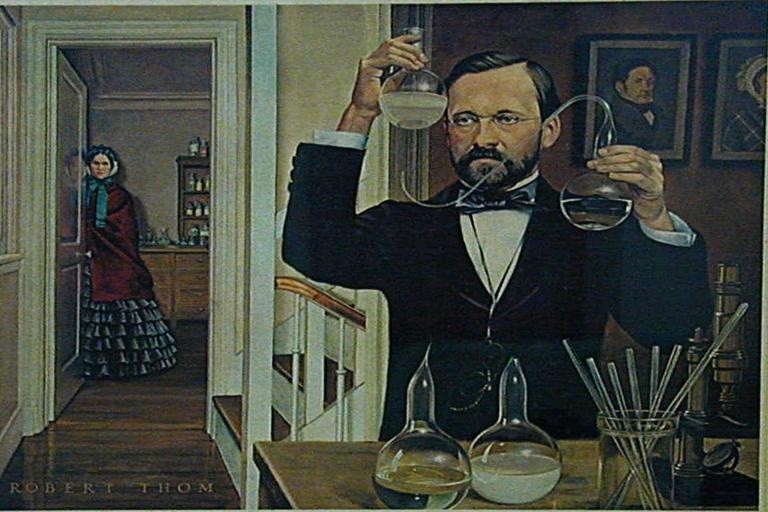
[250,437,761,510]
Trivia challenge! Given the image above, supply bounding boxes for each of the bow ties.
[454,182,537,215]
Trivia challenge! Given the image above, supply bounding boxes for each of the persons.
[74,144,181,383]
[276,31,716,442]
[722,54,766,152]
[602,57,665,153]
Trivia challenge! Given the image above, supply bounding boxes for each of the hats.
[82,144,118,181]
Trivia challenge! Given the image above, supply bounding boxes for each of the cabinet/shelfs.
[140,248,174,327]
[175,155,211,247]
[173,250,210,323]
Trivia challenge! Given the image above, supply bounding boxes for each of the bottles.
[375,351,468,508]
[135,227,173,247]
[558,119,634,232]
[377,28,448,129]
[186,135,210,250]
[470,358,565,505]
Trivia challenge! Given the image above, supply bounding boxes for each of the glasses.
[443,108,545,126]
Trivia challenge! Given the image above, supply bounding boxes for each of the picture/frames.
[575,28,698,169]
[706,32,765,164]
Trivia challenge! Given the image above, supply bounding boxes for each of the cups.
[596,412,676,506]
